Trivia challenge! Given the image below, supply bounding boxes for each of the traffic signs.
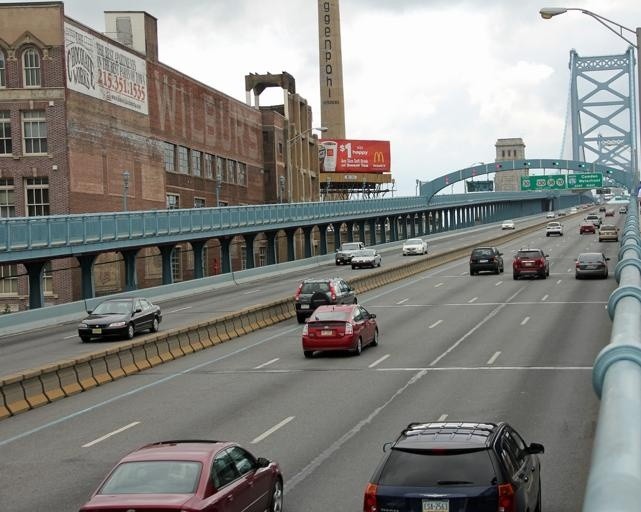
[568,173,604,188]
[521,175,567,190]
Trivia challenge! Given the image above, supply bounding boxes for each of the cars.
[77,439,284,512]
[402,238,428,256]
[350,249,382,269]
[573,252,610,279]
[545,194,629,243]
[77,297,162,344]
[501,219,516,230]
[299,302,378,355]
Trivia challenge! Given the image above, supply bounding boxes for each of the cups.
[318,141,337,172]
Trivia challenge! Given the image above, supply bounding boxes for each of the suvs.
[293,278,358,324]
[360,421,547,511]
[512,247,547,279]
[334,241,365,264]
[468,247,504,275]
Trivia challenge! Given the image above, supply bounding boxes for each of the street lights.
[390,178,395,197]
[361,182,366,199]
[414,178,419,196]
[469,161,485,182]
[120,171,130,211]
[213,174,223,206]
[285,126,328,207]
[278,175,285,202]
[537,4,641,151]
